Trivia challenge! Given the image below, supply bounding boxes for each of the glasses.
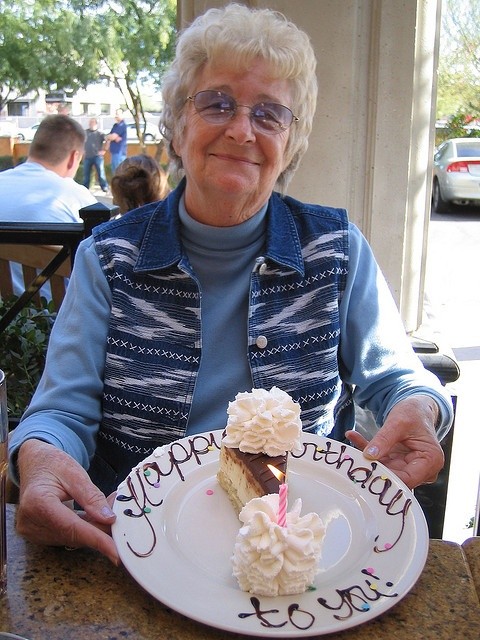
[178,89,300,135]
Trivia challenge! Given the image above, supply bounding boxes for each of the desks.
[0,501,479,639]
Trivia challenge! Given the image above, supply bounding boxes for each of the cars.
[102,121,165,142]
[430,137,480,214]
[17,123,41,141]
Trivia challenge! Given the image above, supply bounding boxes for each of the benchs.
[0,243,75,314]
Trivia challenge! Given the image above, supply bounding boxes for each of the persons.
[5,2,454,567]
[106,108,127,173]
[112,155,168,215]
[82,118,110,191]
[0,114,98,224]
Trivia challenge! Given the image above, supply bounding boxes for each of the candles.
[265,460,291,528]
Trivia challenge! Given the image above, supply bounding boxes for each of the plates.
[110,425,431,635]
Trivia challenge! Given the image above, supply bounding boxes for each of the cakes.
[215,385,307,511]
[231,481,325,596]
